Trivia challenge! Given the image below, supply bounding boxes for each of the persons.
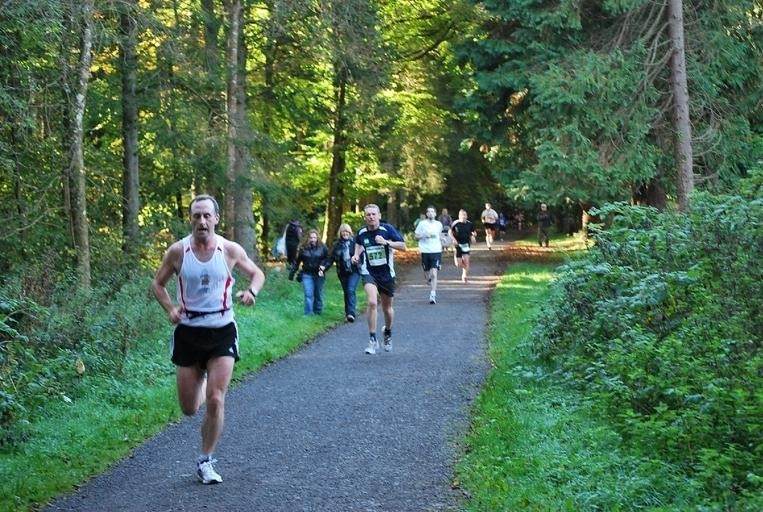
[438,208,452,252]
[447,208,477,284]
[413,211,427,228]
[351,203,407,355]
[414,205,446,305]
[288,229,329,316]
[481,203,556,251]
[292,218,303,264]
[151,193,265,485]
[319,222,360,324]
[286,219,299,269]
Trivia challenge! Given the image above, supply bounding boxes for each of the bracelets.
[385,239,392,246]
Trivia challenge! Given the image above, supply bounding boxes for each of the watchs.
[246,287,258,298]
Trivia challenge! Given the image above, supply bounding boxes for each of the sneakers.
[430,293,436,303]
[347,314,354,322]
[195,462,222,485]
[364,336,378,354]
[381,325,392,351]
